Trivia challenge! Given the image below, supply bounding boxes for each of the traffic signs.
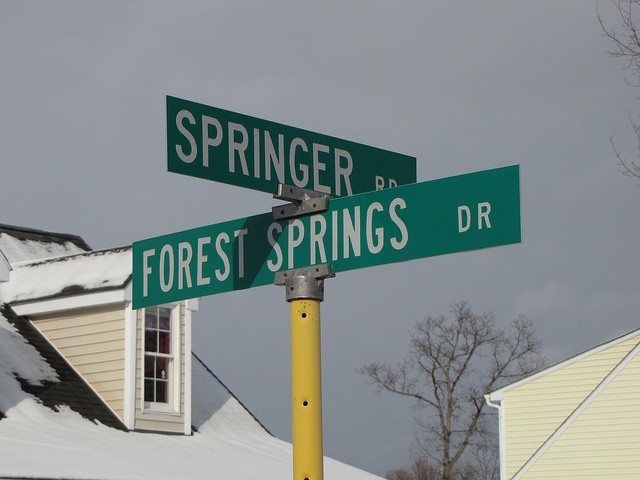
[132,164,521,310]
[166,95,416,198]
